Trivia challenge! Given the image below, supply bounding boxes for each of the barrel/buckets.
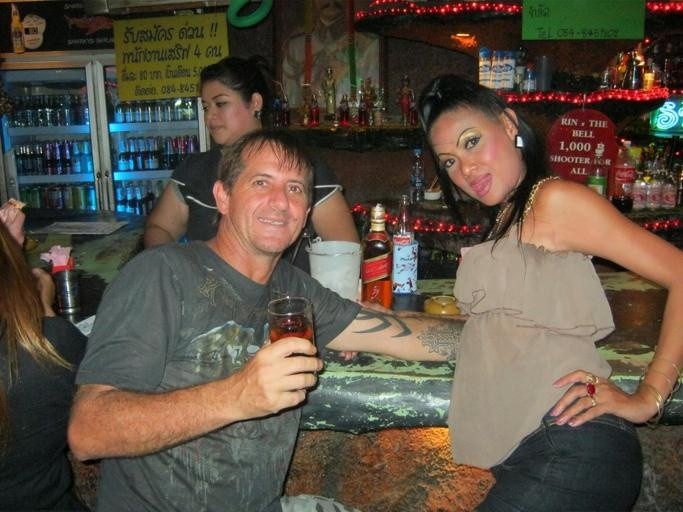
[303,236,365,306]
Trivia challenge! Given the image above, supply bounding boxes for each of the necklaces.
[493,195,513,234]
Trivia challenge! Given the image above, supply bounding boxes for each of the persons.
[67,127,467,512]
[141,55,363,361]
[0,208,26,248]
[415,72,683,512]
[1,215,88,511]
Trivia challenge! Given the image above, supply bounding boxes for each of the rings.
[587,374,597,384]
[585,396,597,407]
[587,383,597,396]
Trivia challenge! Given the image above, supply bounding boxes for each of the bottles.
[588,136,683,213]
[477,47,552,93]
[273,89,417,128]
[390,194,418,294]
[8,89,201,215]
[409,148,425,204]
[602,42,683,89]
[360,202,392,309]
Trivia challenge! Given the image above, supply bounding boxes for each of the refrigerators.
[1,51,212,219]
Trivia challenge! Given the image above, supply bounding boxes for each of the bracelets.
[636,358,682,429]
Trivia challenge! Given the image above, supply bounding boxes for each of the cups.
[266,296,318,378]
[306,241,362,301]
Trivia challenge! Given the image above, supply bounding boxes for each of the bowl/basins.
[423,297,460,316]
[424,189,441,201]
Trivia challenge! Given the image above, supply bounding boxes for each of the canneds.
[20,185,97,212]
[392,239,418,295]
